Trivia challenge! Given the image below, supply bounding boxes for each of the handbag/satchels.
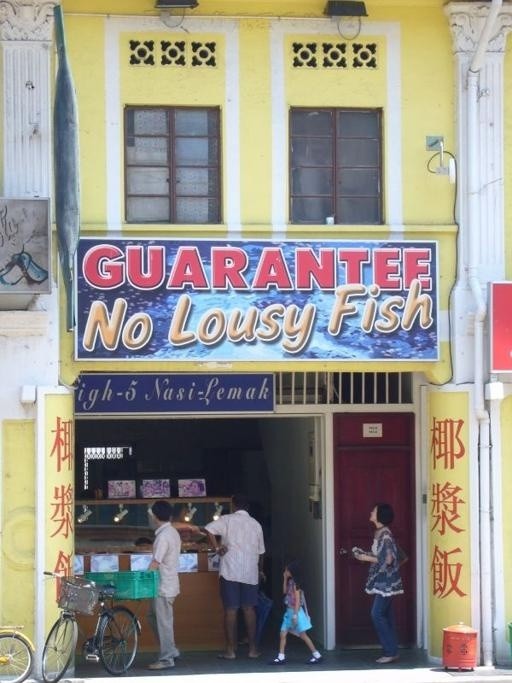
[397,543,409,567]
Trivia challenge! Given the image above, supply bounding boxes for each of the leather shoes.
[143,658,175,670]
[375,653,400,664]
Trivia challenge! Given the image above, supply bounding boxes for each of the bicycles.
[42,571,142,683]
[0,625,36,683]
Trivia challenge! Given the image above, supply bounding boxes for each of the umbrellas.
[254,575,274,650]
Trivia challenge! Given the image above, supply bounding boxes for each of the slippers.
[217,652,236,660]
[247,646,265,658]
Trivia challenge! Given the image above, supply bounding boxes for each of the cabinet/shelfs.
[69,496,236,655]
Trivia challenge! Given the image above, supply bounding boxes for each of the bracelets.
[215,547,220,551]
[292,614,298,619]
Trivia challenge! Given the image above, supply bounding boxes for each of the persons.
[237,632,249,646]
[148,500,183,670]
[133,537,152,545]
[143,481,169,497]
[170,501,200,541]
[110,482,130,496]
[184,481,204,497]
[351,502,410,664]
[267,557,324,665]
[203,489,268,662]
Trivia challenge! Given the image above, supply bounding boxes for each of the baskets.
[58,576,97,615]
[84,569,159,601]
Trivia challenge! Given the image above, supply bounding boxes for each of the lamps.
[77,503,129,524]
[183,501,223,522]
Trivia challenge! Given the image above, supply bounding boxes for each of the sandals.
[305,655,323,664]
[269,656,288,665]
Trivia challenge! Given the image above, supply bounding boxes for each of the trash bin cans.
[441,622,478,672]
